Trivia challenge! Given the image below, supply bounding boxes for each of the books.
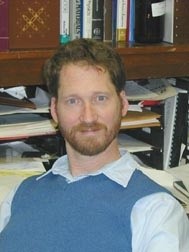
[60,0,169,46]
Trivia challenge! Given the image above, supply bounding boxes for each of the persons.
[1,37,189,252]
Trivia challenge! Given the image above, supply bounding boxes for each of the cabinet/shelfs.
[0,0,188,215]
